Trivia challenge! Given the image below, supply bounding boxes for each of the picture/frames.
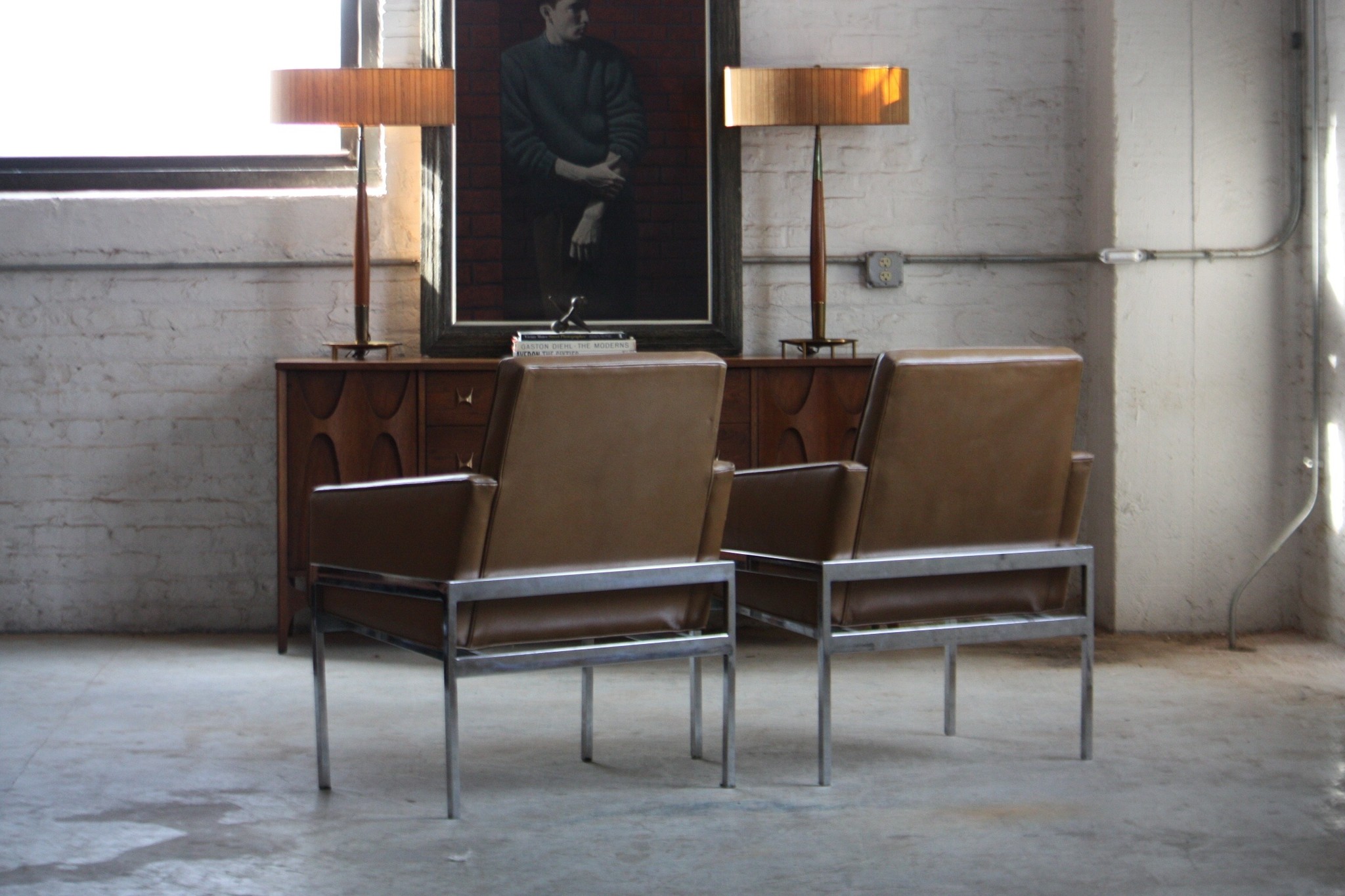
[421,0,743,356]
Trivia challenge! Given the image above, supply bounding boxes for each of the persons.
[499,0,655,323]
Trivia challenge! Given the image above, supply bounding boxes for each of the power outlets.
[866,250,902,287]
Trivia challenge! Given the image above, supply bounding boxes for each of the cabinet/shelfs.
[274,350,880,652]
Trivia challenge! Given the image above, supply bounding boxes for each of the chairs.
[306,350,736,819]
[692,347,1094,785]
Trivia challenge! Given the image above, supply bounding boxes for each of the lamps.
[270,68,457,359]
[723,65,910,356]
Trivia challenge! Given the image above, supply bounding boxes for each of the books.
[512,328,636,355]
